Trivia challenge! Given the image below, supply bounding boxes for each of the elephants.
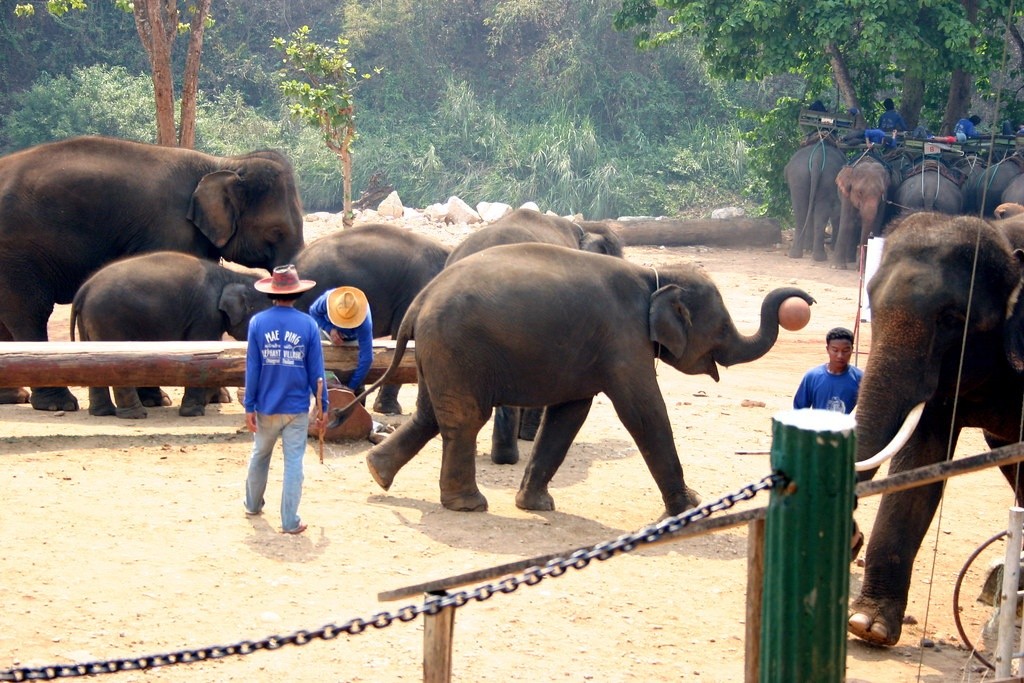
[781,135,1023,270]
[288,210,819,517]
[847,202,1024,647]
[1,135,304,419]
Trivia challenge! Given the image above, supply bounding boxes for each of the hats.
[254,264,317,294]
[883,136,892,147]
[327,286,368,329]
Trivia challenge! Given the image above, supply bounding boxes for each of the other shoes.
[283,523,307,533]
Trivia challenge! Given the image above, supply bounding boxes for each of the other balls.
[779,296,811,331]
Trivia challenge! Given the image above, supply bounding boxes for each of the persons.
[242,264,329,535]
[839,129,898,150]
[309,285,373,408]
[877,99,908,132]
[793,327,864,562]
[908,125,966,144]
[951,115,989,137]
